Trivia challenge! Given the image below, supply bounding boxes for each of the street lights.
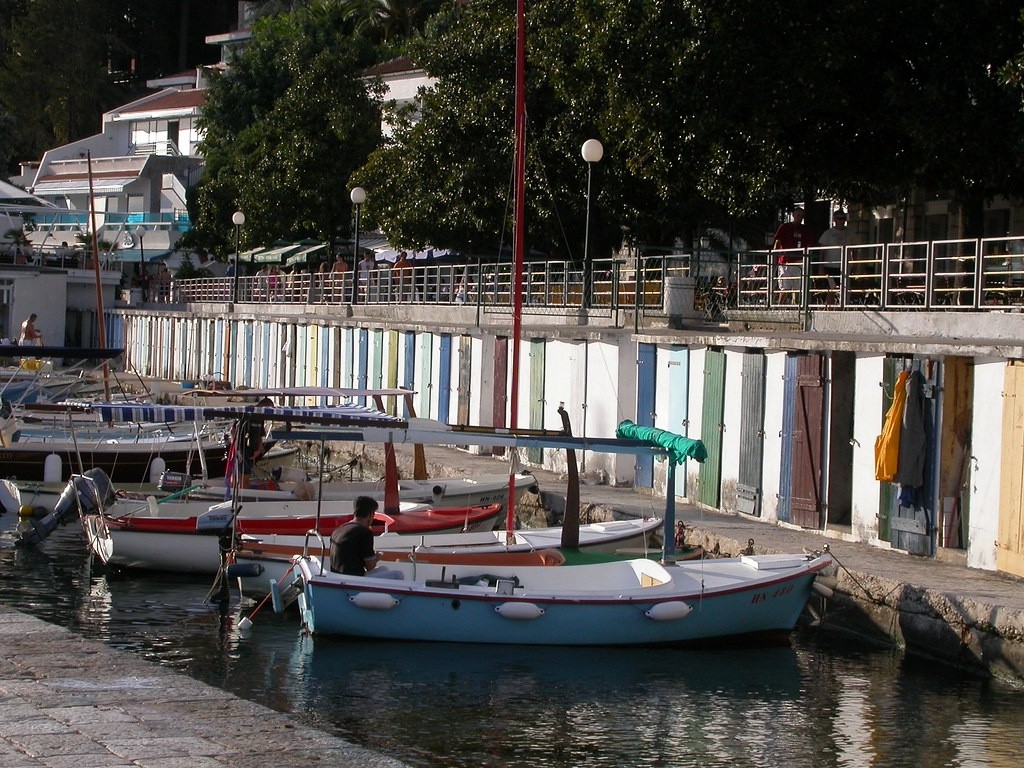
[135,225,147,301]
[232,212,245,304]
[580,139,604,309]
[350,187,366,304]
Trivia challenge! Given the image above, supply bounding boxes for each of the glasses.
[834,216,847,221]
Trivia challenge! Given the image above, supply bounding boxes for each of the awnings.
[254,245,303,263]
[228,246,265,262]
[286,245,326,267]
[33,179,138,195]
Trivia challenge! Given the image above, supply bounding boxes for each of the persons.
[18,313,43,359]
[224,259,243,303]
[773,208,809,305]
[329,496,382,577]
[393,251,412,305]
[22,241,32,263]
[56,242,68,261]
[357,249,374,305]
[818,209,851,311]
[254,253,348,301]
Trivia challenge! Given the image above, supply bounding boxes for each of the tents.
[447,239,570,262]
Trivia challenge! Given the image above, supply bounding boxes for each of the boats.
[270,419,834,645]
[0,345,705,608]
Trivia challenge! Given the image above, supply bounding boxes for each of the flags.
[224,427,239,501]
